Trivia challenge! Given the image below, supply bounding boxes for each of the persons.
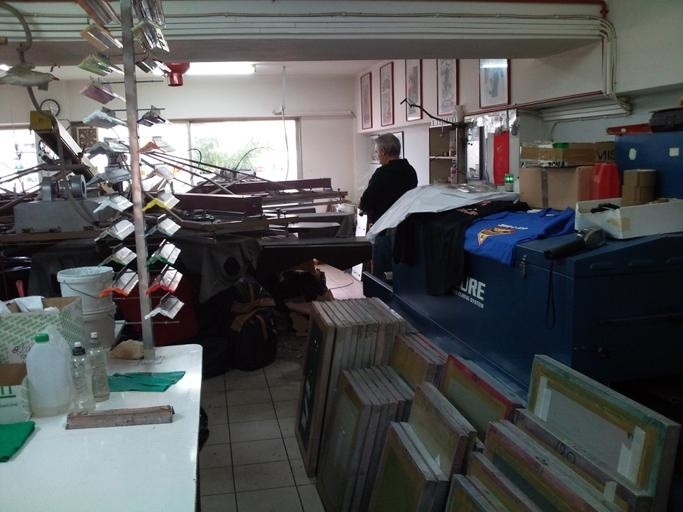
[357,133,418,239]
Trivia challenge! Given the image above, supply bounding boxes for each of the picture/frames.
[359,58,510,130]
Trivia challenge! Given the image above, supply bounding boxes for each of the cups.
[503,172,514,192]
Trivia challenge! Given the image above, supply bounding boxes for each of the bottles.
[66,340,96,413]
[86,331,110,403]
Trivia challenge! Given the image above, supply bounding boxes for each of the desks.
[0,343,204,512]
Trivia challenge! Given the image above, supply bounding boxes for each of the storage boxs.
[516,141,614,213]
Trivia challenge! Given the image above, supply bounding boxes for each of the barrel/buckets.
[26,324,73,419]
[590,162,620,200]
[57,266,117,352]
